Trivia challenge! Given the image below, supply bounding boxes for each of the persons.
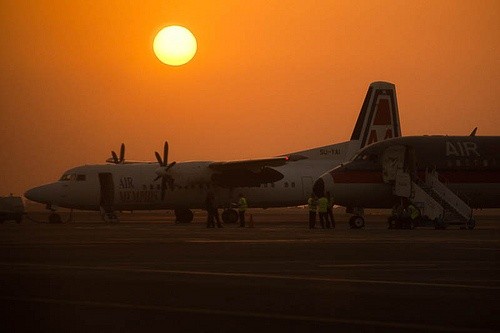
[407,205,418,229]
[388,203,402,229]
[205,191,224,229]
[308,192,335,229]
[236,193,248,225]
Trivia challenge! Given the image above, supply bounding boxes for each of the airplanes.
[310,128,500,232]
[23,80,403,224]
